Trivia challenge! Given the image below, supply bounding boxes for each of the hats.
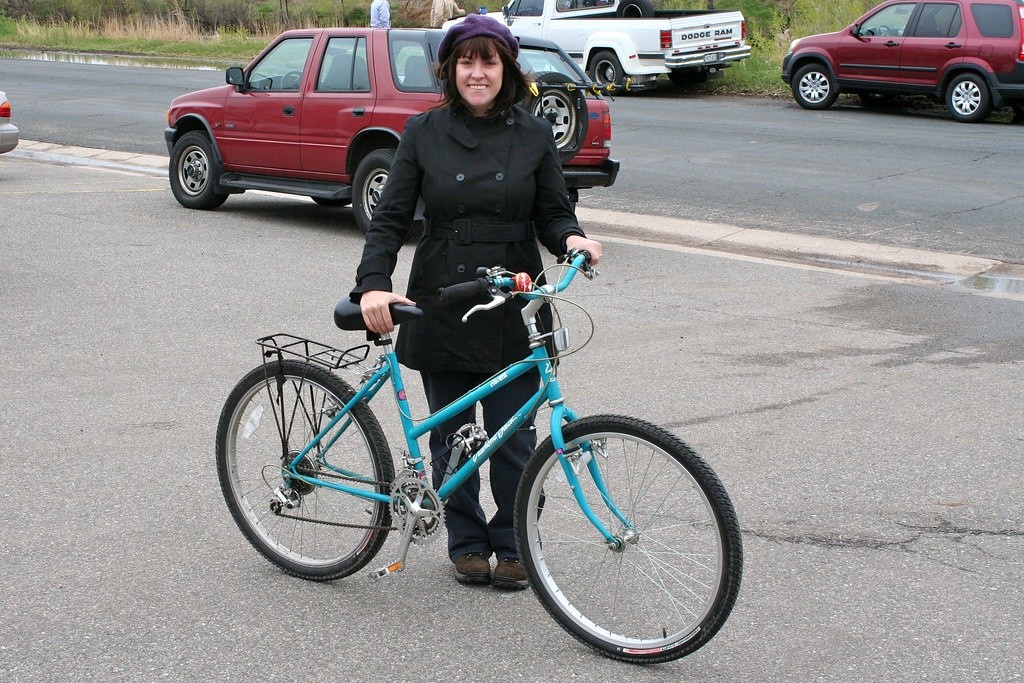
[438,13,519,67]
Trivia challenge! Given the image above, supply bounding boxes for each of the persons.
[371,0,390,28]
[350,14,602,591]
[430,0,465,27]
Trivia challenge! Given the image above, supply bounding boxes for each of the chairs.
[915,12,938,37]
[402,54,432,86]
[316,54,369,92]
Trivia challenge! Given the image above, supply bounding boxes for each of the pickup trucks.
[442,0,756,100]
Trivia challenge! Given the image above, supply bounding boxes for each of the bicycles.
[216,249,745,666]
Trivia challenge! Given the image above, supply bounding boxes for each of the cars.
[0,90,19,155]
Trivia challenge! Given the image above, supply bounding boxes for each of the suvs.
[780,0,1024,124]
[165,22,619,244]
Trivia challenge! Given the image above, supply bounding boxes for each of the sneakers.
[453,553,490,583]
[492,557,528,590]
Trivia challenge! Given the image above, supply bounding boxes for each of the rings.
[600,254,602,257]
